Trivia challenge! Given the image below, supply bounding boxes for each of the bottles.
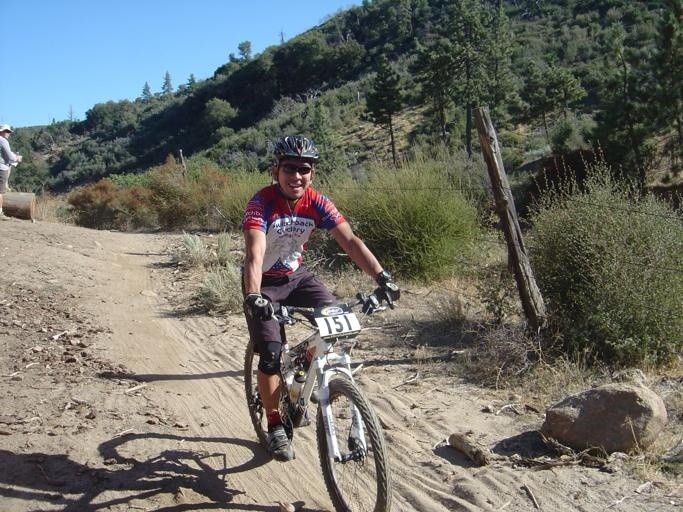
[288,370,306,404]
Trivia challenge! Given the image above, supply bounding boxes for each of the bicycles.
[244,285,400,508]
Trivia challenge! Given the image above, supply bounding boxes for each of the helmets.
[274,135,319,162]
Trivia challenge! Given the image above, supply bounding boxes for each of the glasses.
[279,164,312,174]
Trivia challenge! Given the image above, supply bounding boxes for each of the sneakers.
[268,422,293,462]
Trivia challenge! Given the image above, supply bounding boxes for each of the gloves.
[244,294,274,321]
[376,270,400,309]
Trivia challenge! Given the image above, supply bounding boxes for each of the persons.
[240,133,401,461]
[0,123,22,221]
